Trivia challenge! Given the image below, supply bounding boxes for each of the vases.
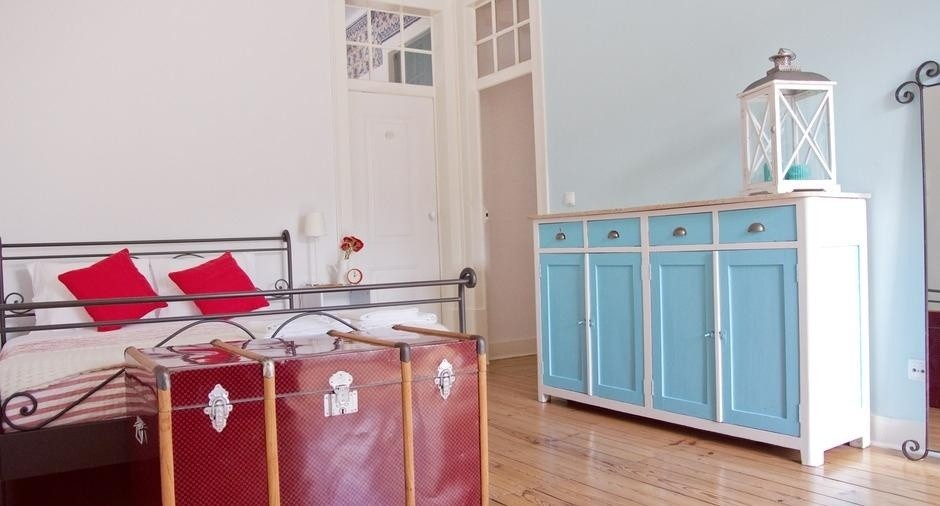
[337,259,350,283]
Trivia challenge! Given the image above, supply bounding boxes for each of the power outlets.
[907,359,925,382]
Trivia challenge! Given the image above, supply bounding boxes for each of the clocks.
[346,268,363,284]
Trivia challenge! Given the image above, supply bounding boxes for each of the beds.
[2,228,477,506]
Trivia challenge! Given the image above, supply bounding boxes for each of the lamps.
[304,212,326,287]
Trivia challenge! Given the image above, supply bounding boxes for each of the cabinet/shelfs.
[123,322,490,506]
[648,205,801,438]
[539,216,645,407]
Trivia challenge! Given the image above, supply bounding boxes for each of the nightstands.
[299,285,373,310]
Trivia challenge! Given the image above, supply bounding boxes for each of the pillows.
[168,250,269,322]
[58,248,168,332]
[24,258,157,334]
[149,258,276,318]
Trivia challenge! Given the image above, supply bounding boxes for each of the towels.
[266,307,437,339]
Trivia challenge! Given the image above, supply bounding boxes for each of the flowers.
[340,235,364,259]
